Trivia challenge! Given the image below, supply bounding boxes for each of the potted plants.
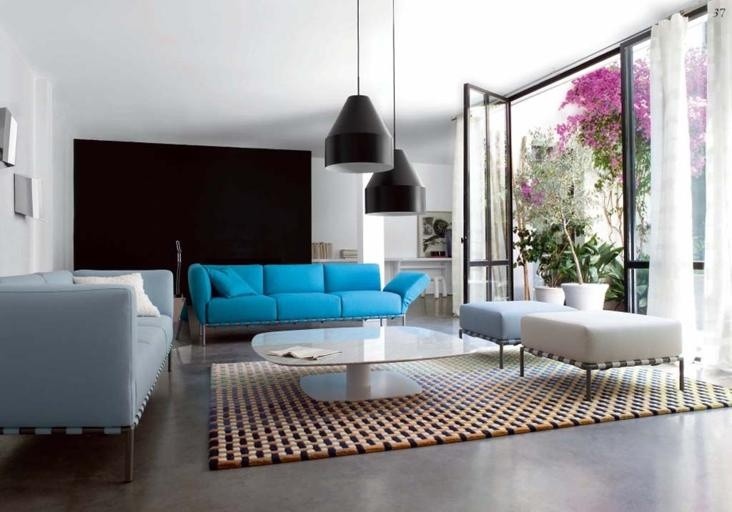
[510,220,625,313]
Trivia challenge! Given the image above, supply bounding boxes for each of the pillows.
[71,272,160,316]
[207,266,257,298]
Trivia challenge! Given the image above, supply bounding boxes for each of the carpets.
[199,349,732,471]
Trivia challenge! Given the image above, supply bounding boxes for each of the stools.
[453,298,683,398]
[420,276,447,298]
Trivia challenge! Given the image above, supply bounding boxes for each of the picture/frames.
[416,211,452,258]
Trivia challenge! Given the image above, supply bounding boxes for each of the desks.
[389,257,452,296]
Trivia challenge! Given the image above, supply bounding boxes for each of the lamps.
[322,0,427,218]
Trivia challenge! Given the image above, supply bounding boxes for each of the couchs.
[186,261,429,348]
[0,269,174,483]
